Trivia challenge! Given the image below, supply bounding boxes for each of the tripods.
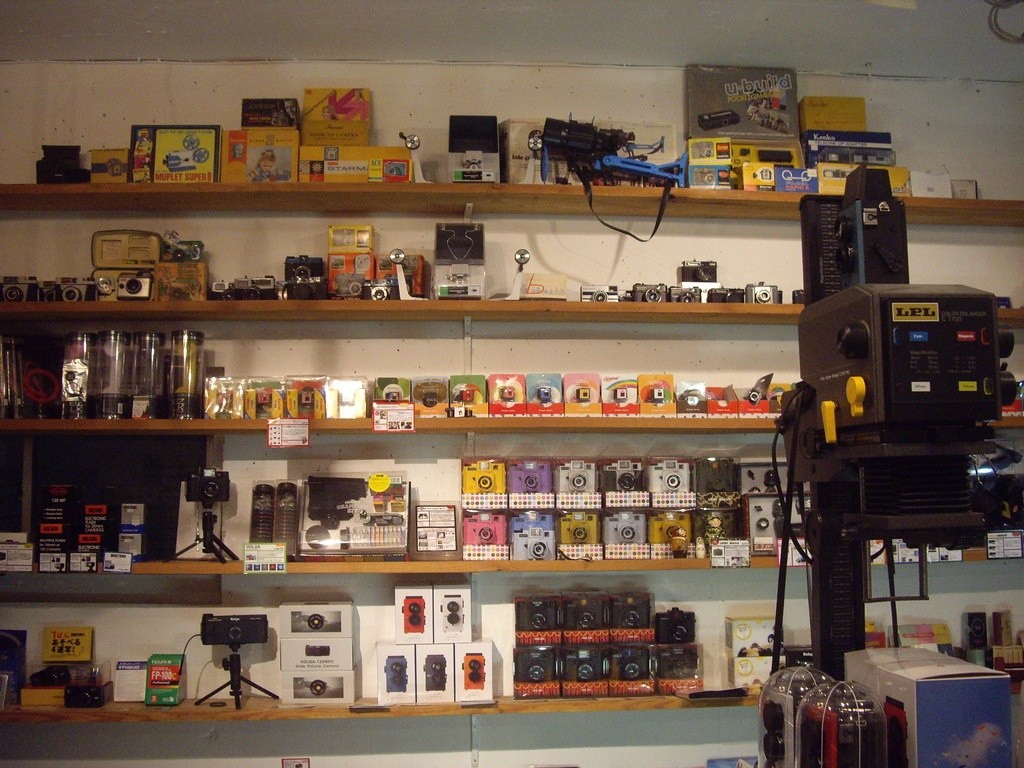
[194,644,279,710]
[164,502,238,565]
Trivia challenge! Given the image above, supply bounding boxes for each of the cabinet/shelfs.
[0,172,1022,726]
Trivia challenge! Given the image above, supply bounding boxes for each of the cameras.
[186,468,230,502]
[160,240,204,263]
[512,591,698,684]
[206,248,424,301]
[461,458,748,563]
[201,614,268,645]
[792,290,804,304]
[384,595,486,692]
[64,686,104,709]
[580,261,783,304]
[0,272,154,303]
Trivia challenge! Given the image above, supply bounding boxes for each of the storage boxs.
[21,658,111,688]
[19,683,65,706]
[36,483,147,562]
[496,117,557,184]
[681,61,978,200]
[247,455,811,562]
[275,582,792,707]
[325,225,425,300]
[842,647,1015,768]
[86,85,414,184]
